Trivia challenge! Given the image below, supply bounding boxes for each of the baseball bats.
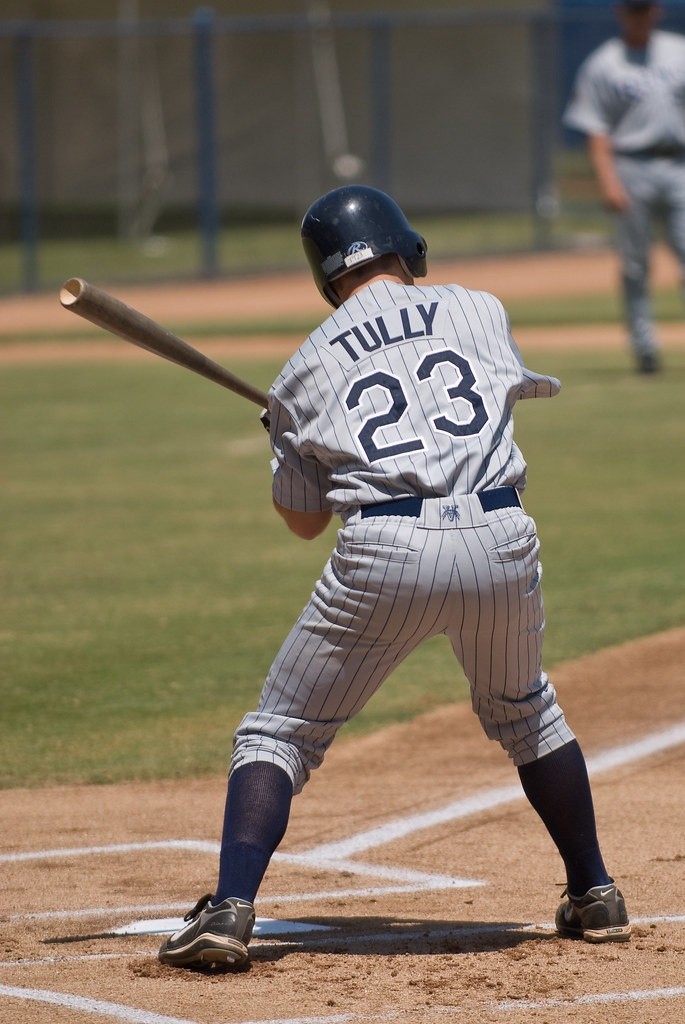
[59,278,268,410]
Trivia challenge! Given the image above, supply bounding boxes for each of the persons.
[159,185,634,971]
[562,0,684,376]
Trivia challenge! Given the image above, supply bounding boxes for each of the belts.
[360,486,522,520]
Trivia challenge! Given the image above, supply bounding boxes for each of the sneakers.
[157,893,257,970]
[555,875,631,943]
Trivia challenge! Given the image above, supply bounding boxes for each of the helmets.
[301,186,428,307]
